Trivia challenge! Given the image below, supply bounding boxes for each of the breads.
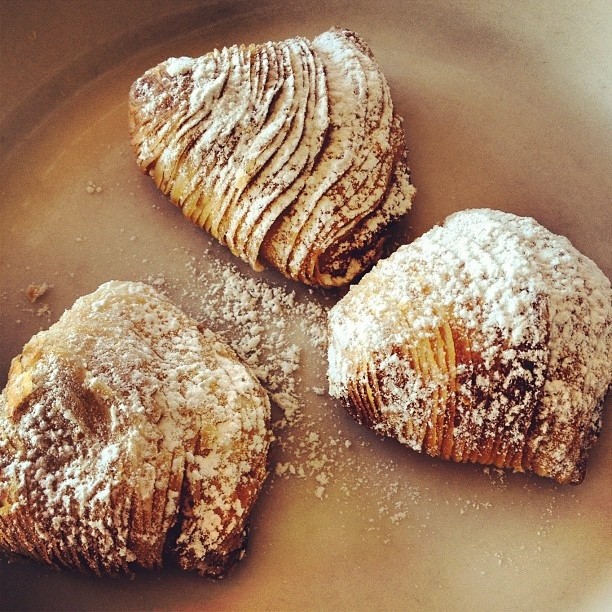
[326,205,612,485]
[0,279,270,581]
[128,25,418,290]
